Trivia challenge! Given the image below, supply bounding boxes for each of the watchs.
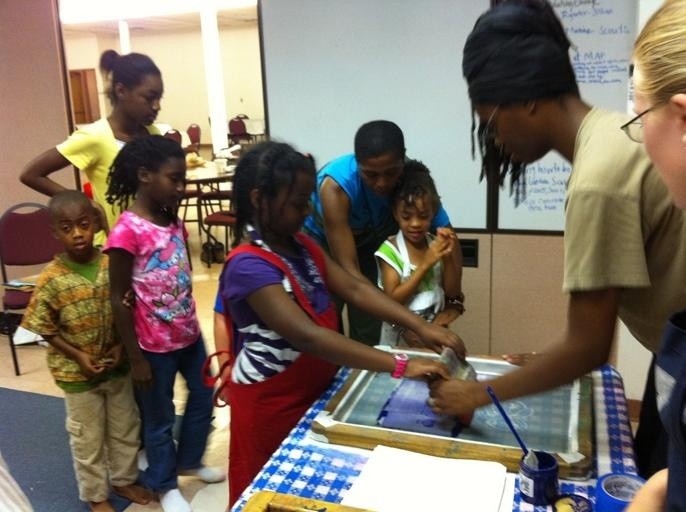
[390,352,409,379]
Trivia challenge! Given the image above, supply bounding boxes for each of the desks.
[225,341,641,512]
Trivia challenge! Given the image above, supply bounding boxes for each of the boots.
[201,242,216,264]
[213,242,224,263]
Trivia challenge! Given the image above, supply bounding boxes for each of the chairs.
[162,110,257,268]
[0,200,70,376]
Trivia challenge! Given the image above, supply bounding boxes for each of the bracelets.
[447,292,465,301]
[447,300,466,314]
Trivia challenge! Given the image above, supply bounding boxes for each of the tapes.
[595,473,648,512]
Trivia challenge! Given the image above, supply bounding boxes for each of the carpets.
[0,383,217,511]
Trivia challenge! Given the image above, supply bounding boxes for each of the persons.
[371,157,463,350]
[423,0,686,484]
[212,284,233,383]
[219,138,470,510]
[617,1,686,512]
[297,116,467,346]
[98,131,230,512]
[17,47,181,474]
[15,189,153,512]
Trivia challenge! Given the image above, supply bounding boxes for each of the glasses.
[620,104,659,142]
[478,111,501,141]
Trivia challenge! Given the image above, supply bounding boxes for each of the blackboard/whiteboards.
[258,1,639,236]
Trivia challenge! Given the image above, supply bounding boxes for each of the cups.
[519,451,561,506]
[214,158,228,174]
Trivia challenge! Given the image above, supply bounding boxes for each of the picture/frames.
[309,342,599,481]
[243,481,370,512]
